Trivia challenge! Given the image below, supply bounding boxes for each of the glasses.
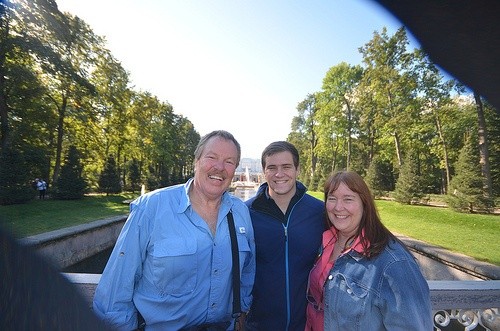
[306,265,325,311]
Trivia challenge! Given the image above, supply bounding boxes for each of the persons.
[92,129,257,331]
[243,141,333,331]
[37,177,47,200]
[303,169,434,331]
